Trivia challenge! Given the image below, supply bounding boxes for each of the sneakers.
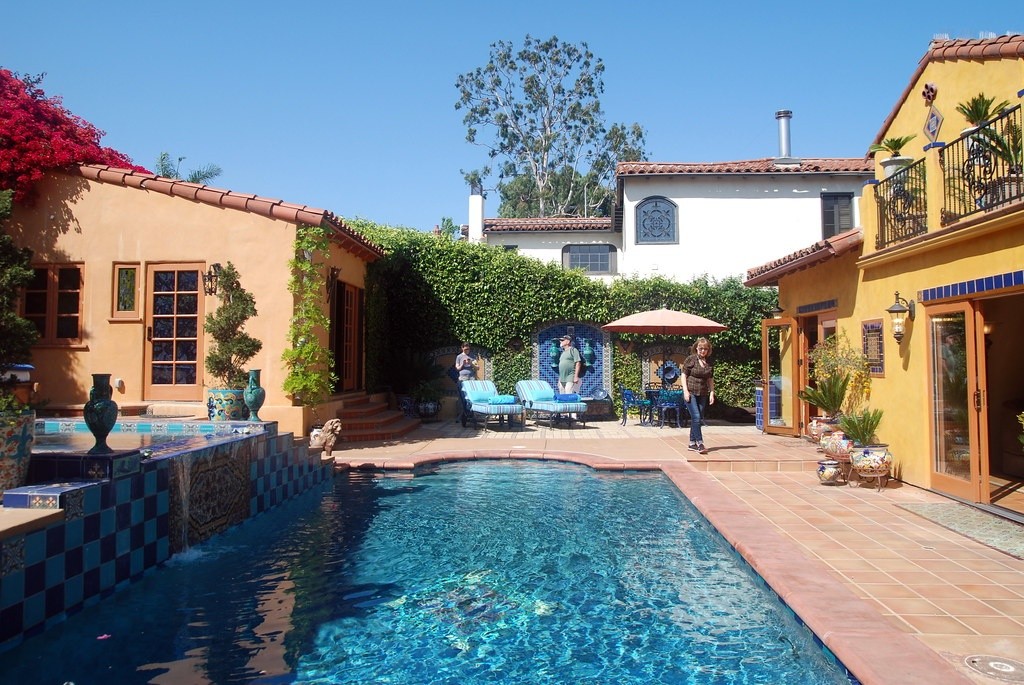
[698,444,708,453]
[688,444,699,451]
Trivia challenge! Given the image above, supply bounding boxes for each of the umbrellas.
[601,304,728,390]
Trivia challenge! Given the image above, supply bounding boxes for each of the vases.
[397,394,417,419]
[818,429,881,463]
[83,374,119,453]
[243,369,267,424]
[817,461,842,484]
[808,416,835,445]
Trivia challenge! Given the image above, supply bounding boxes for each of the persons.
[558,335,581,426]
[455,342,479,421]
[936,333,957,410]
[681,337,714,453]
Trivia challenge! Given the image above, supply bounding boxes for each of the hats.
[559,335,572,341]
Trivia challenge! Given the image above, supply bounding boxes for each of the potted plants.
[280,224,338,445]
[419,378,442,419]
[0,187,33,506]
[869,93,1024,231]
[835,407,892,477]
[796,363,849,440]
[201,262,262,422]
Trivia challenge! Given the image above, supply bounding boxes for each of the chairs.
[457,378,526,430]
[515,379,588,429]
[618,382,707,430]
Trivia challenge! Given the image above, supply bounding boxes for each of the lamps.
[885,290,915,344]
[325,265,342,301]
[201,263,222,296]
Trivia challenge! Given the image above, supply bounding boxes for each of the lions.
[313,418,341,457]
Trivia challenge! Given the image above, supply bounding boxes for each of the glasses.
[698,348,709,352]
